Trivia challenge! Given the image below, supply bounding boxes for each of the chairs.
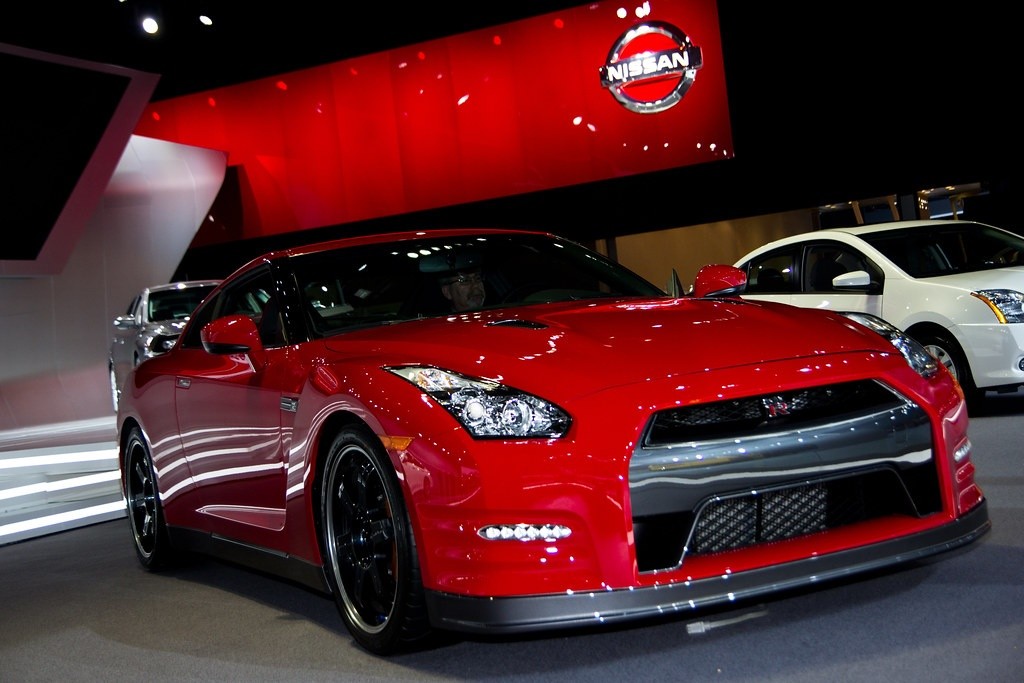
[259,286,326,349]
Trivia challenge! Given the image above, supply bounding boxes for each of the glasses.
[444,274,483,286]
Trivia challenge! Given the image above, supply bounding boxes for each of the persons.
[442,259,485,312]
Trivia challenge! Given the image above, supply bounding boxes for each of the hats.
[435,250,481,276]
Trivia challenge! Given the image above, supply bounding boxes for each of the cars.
[690,219,1023,405]
[107,281,272,413]
[117,226,992,653]
